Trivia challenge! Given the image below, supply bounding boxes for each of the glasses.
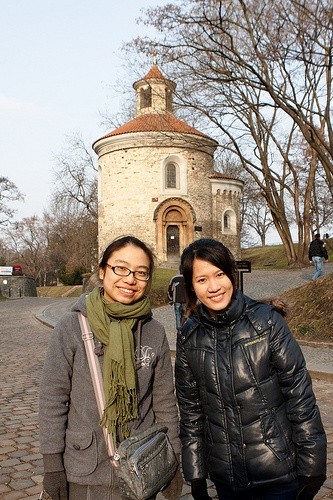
[105,263,152,282]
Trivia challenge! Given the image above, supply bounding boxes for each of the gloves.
[296,475,325,500]
[43,471,68,500]
[161,465,183,500]
[190,478,213,500]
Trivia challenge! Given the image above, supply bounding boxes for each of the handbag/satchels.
[113,425,178,500]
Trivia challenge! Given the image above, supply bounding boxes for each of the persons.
[167,264,191,332]
[309,234,329,282]
[39,236,183,500]
[175,238,327,500]
